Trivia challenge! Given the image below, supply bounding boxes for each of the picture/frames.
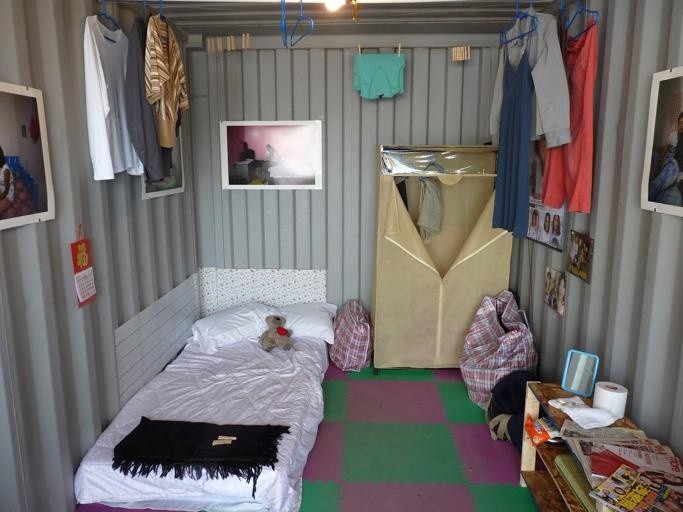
[219,120,324,190]
[0,80,56,232]
[140,122,185,199]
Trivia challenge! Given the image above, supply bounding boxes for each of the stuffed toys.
[258,316,292,352]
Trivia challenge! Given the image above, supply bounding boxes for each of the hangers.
[279,0,288,47]
[387,148,413,178]
[291,0,316,47]
[419,150,446,182]
[89,0,166,43]
[499,0,600,45]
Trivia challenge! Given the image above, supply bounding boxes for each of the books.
[558,418,683,511]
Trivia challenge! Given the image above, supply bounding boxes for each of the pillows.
[276,301,337,345]
[191,301,275,355]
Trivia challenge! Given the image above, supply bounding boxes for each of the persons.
[261,144,281,184]
[527,210,561,250]
[656,112,683,207]
[543,270,567,316]
[0,145,15,213]
[570,235,590,274]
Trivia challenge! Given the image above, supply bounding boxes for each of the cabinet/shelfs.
[518,381,683,511]
[369,144,512,376]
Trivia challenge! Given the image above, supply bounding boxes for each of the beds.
[74,305,329,512]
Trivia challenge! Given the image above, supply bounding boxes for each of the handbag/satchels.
[328,299,373,372]
[459,289,538,409]
[485,369,541,449]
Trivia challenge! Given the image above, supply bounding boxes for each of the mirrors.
[560,350,599,399]
